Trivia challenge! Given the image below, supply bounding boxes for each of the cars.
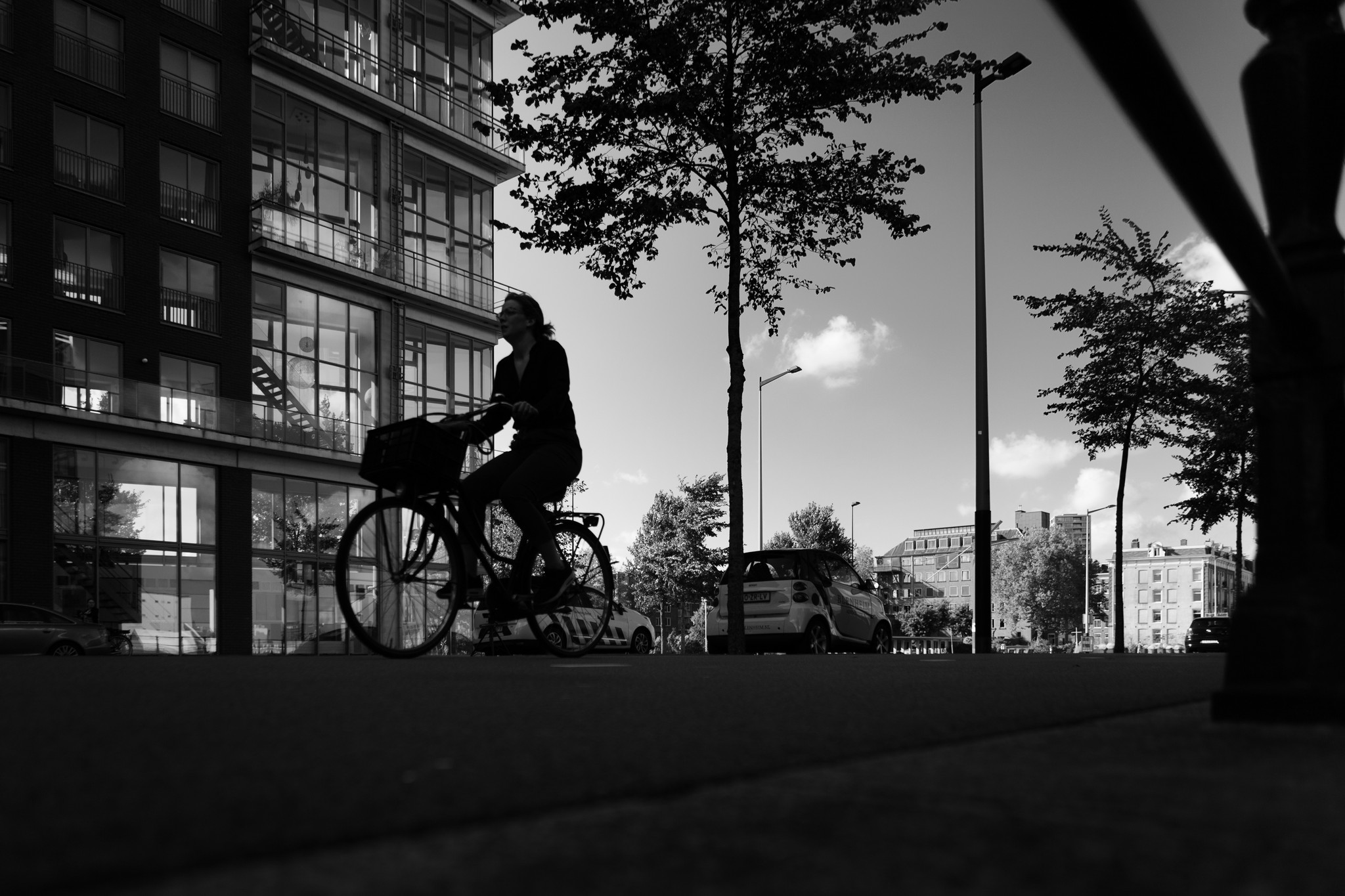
[469,576,656,657]
[0,602,108,659]
[705,548,893,656]
[289,626,377,655]
[1185,617,1229,654]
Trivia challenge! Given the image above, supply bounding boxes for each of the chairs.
[748,563,778,580]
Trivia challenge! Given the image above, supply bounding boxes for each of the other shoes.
[537,562,573,600]
[436,577,482,599]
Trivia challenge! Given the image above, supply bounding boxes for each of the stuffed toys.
[784,569,794,577]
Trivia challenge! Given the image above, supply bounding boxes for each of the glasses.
[496,307,514,318]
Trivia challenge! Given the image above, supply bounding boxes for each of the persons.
[1136,642,1143,653]
[80,599,98,623]
[436,292,583,604]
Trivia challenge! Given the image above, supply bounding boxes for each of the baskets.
[357,413,464,493]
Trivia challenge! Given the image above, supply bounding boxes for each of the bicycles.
[335,393,626,660]
[71,613,133,657]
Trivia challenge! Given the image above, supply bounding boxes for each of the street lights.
[759,365,802,551]
[571,478,580,573]
[852,501,861,570]
[1084,505,1117,654]
[1214,552,1237,625]
